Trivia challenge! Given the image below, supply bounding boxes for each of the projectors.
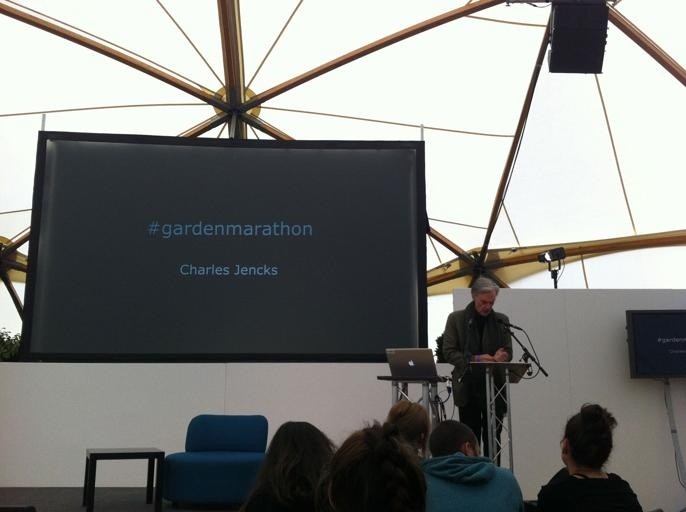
[545,3,610,75]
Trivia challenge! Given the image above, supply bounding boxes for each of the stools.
[82,448,165,512]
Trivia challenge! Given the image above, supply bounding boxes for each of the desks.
[376,376,445,461]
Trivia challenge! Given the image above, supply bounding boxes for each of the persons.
[538,404,644,512]
[329,399,426,512]
[419,417,524,512]
[444,275,512,468]
[237,421,334,512]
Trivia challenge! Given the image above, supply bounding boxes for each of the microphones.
[496,318,522,331]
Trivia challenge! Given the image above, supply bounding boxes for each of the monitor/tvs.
[624,309,686,378]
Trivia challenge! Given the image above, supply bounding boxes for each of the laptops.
[385,348,437,376]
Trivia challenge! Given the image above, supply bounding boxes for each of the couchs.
[162,415,274,512]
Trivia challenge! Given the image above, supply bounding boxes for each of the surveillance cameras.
[538,247,566,263]
